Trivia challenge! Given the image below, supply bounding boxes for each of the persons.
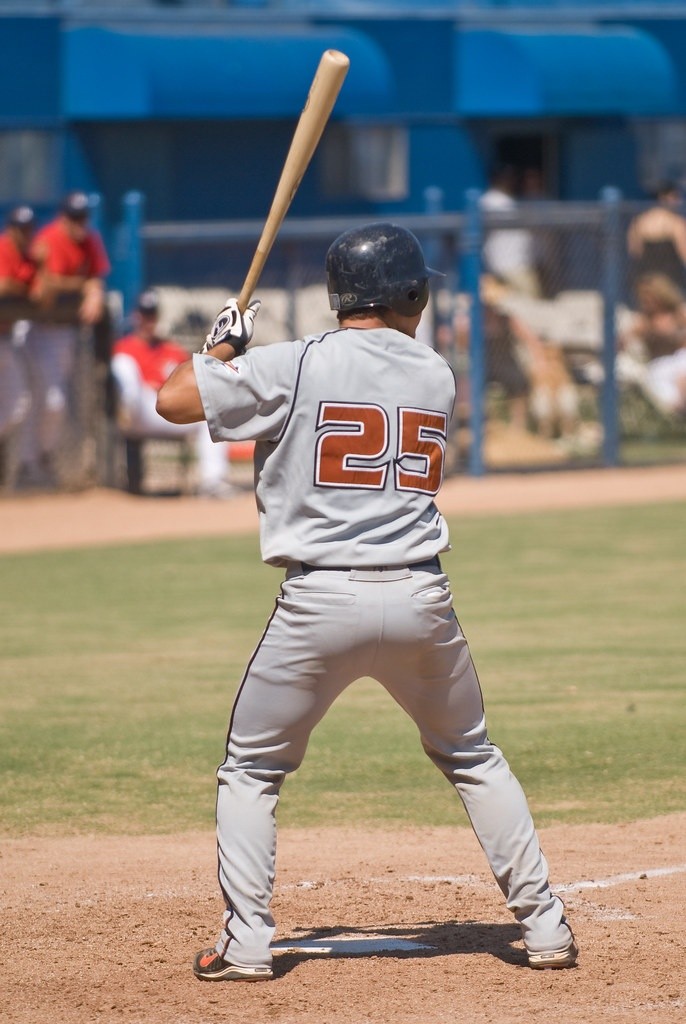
[0,192,241,502]
[475,158,686,440]
[156,225,581,983]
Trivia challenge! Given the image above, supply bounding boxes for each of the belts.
[301,553,441,573]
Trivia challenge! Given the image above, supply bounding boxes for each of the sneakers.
[529,941,577,968]
[193,948,273,982]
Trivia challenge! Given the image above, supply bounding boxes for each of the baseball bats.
[234,45,352,315]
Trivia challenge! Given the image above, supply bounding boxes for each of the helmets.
[138,291,161,317]
[64,191,90,219]
[9,205,35,233]
[324,223,445,316]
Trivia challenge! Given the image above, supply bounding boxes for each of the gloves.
[197,296,261,357]
